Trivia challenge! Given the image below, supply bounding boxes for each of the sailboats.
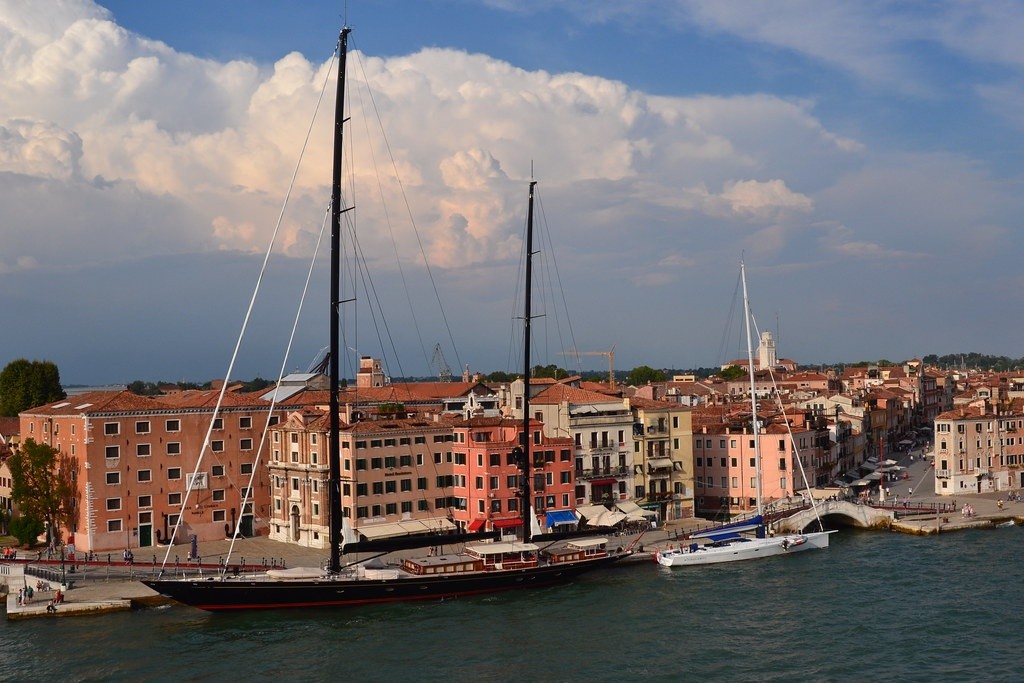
[128,17,650,613]
[647,250,840,567]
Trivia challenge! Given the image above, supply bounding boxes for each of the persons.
[4,547,132,610]
[187,551,194,567]
[860,439,1021,516]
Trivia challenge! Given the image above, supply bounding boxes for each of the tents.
[795,426,932,499]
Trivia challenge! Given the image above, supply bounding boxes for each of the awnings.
[468,519,523,530]
[546,499,659,530]
